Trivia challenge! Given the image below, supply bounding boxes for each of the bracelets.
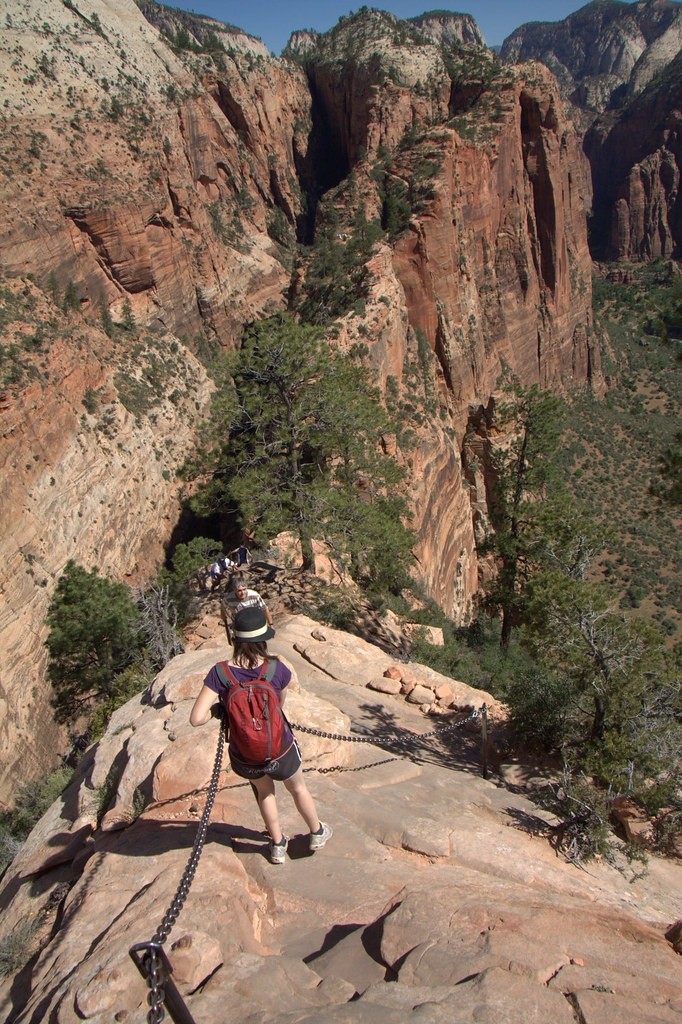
[269,624,273,625]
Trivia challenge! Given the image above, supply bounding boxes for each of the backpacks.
[216,657,287,766]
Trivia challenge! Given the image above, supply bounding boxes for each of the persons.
[209,540,253,594]
[226,579,275,646]
[189,606,333,865]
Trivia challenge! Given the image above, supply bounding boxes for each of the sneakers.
[309,821,333,850]
[270,833,288,864]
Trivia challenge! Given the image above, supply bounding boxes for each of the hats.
[233,608,275,641]
